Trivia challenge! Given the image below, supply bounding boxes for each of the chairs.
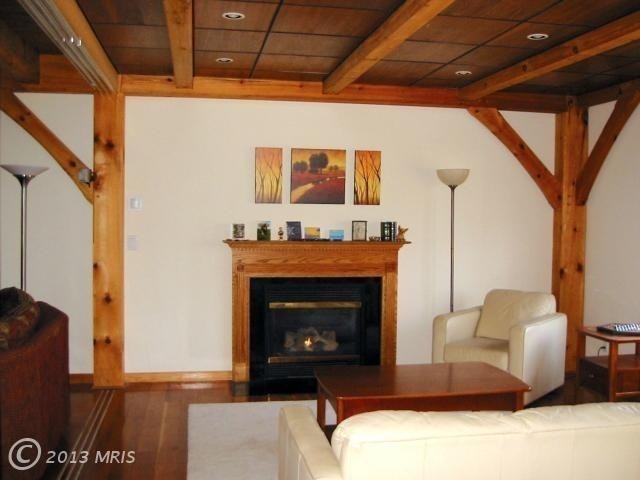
[432,289,568,406]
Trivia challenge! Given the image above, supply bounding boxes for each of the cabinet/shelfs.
[576,326,640,401]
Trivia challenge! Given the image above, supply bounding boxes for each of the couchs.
[279,402,639,480]
[1,287,70,480]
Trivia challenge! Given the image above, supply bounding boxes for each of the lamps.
[436,169,470,312]
[0,165,50,292]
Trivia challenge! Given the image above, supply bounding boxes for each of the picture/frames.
[352,221,366,241]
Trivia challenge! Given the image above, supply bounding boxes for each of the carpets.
[187,398,336,480]
